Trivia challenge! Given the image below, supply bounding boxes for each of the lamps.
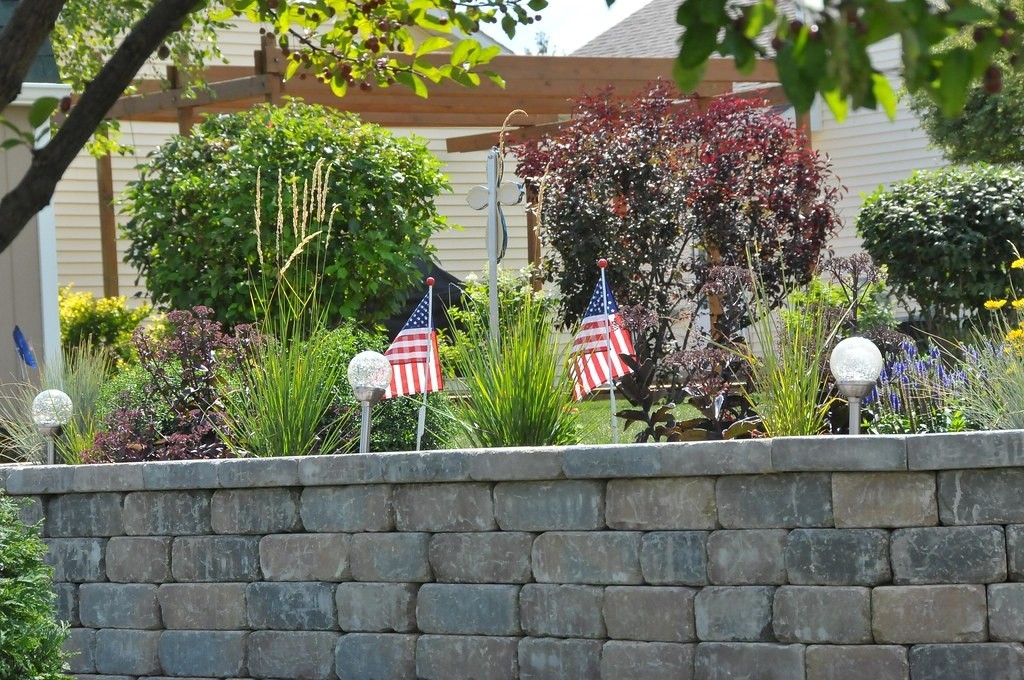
[830,337,883,382]
[31,390,73,428]
[348,350,392,390]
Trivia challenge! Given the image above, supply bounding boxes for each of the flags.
[565,277,639,402]
[382,288,444,400]
